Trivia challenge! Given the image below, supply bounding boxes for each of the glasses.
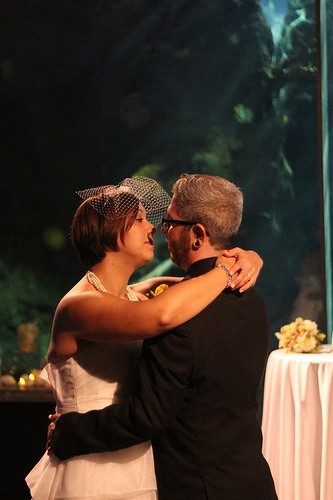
[161,217,210,237]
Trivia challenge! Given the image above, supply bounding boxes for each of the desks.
[261,345,333,500]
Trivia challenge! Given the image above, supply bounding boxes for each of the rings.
[248,274,252,280]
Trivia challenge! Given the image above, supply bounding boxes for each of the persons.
[24,176,263,500]
[49,172,280,500]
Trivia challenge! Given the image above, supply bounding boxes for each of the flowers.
[274,317,326,354]
[149,284,169,297]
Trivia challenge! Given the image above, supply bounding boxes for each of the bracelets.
[215,263,232,288]
